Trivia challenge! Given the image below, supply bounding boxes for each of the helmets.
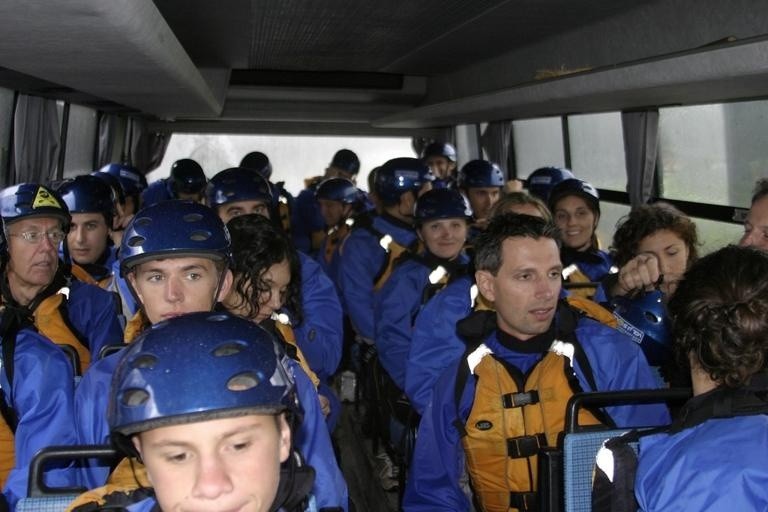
[458,161,504,188]
[315,178,360,205]
[333,150,359,175]
[612,291,678,365]
[57,163,148,225]
[526,167,600,229]
[108,310,305,457]
[207,167,273,215]
[1,181,71,235]
[120,200,233,278]
[170,159,207,194]
[414,188,475,226]
[241,152,272,178]
[420,141,457,163]
[376,157,435,199]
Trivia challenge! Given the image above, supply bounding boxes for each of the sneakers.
[339,375,362,403]
[377,454,401,492]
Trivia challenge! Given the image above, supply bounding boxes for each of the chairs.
[8,280,692,511]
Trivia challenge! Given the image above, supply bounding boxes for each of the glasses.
[8,230,66,244]
